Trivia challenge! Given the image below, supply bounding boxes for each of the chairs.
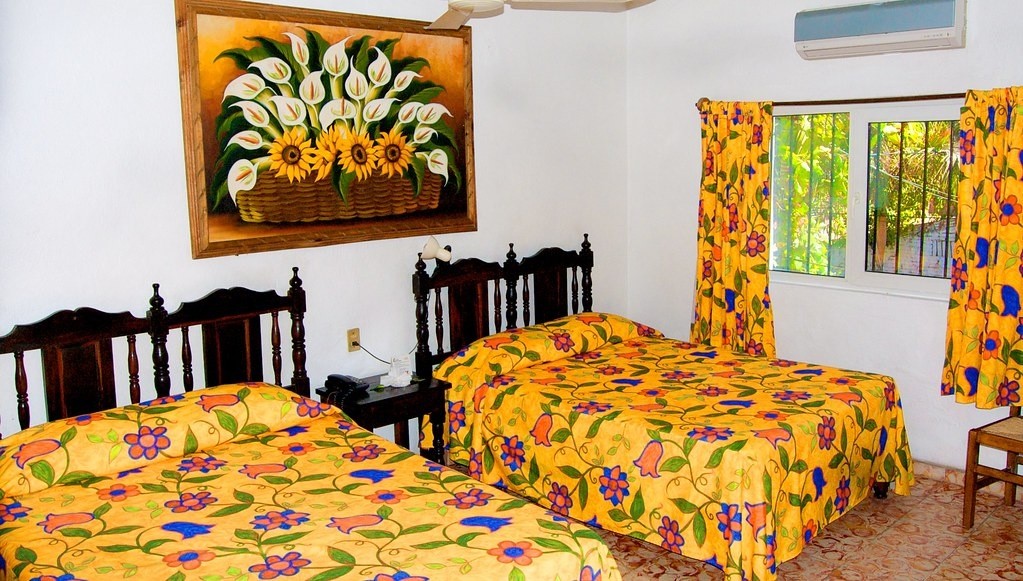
[962,407,1023,528]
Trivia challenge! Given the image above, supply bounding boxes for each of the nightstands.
[315,371,452,462]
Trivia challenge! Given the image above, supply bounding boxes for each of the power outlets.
[347,328,361,351]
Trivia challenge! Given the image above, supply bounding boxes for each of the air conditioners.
[793,0,966,61]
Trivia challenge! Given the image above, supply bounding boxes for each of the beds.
[411,231,916,581]
[0,267,622,581]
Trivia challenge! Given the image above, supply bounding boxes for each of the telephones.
[325,374,370,397]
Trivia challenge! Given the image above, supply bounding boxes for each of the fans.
[424,0,659,29]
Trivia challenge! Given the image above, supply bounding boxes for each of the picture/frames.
[172,0,479,259]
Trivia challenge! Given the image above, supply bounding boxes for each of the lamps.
[418,236,451,262]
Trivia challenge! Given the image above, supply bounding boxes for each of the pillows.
[435,312,662,396]
[0,382,354,499]
